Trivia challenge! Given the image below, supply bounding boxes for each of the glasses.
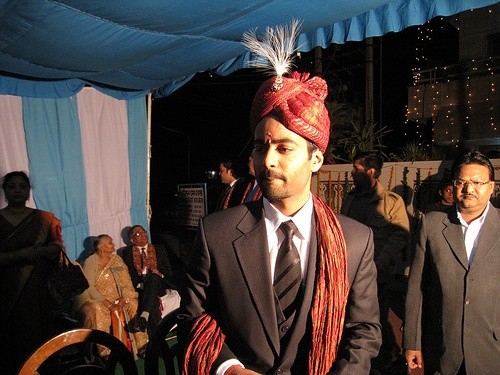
[452,180,490,188]
[131,231,146,238]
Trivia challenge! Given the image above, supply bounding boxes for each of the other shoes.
[101,355,110,364]
[133,317,146,332]
[139,348,146,359]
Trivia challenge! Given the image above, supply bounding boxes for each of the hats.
[242,18,330,155]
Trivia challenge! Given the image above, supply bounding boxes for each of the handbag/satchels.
[48,243,89,296]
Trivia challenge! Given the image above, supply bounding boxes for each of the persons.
[0,171,63,375]
[401,152,500,375]
[239,149,264,205]
[174,71,383,375]
[56,244,96,330]
[213,159,247,211]
[340,148,411,323]
[83,235,150,361]
[123,226,172,336]
[425,181,455,213]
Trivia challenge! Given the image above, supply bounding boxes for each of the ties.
[141,248,146,269]
[273,221,302,321]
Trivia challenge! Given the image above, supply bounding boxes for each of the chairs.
[144,307,187,375]
[18,328,138,375]
